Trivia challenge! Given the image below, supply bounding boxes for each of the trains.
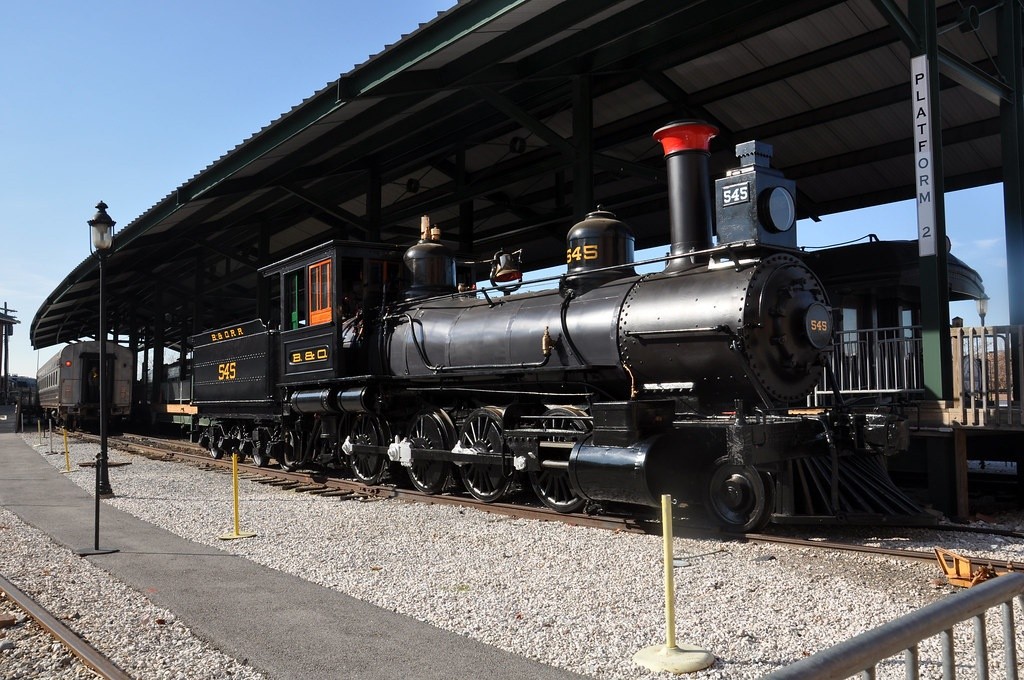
[36,339,135,435]
[188,116,916,536]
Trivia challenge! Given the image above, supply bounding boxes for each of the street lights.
[86,199,117,495]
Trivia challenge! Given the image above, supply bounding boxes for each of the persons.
[88,365,101,405]
